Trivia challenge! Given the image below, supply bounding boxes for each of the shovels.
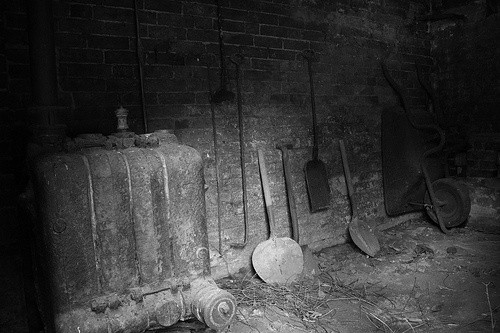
[339,140,381,258]
[247,149,305,284]
[301,49,332,213]
